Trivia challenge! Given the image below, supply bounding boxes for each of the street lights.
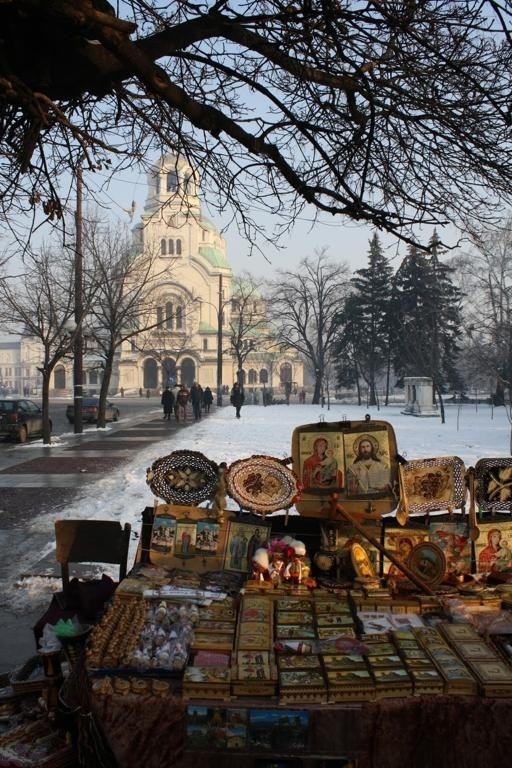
[193,274,238,406]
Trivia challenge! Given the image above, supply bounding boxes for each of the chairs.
[34,520,131,654]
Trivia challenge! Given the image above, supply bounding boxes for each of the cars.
[0,398,52,443]
[66,396,119,423]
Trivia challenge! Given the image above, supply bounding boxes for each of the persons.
[346,440,391,496]
[321,396,326,407]
[155,382,214,425]
[292,387,306,405]
[388,537,414,575]
[180,527,191,554]
[302,438,342,488]
[230,382,245,418]
[221,384,229,395]
[478,528,512,573]
[146,390,150,399]
[139,388,143,397]
[120,387,126,397]
[229,527,261,571]
